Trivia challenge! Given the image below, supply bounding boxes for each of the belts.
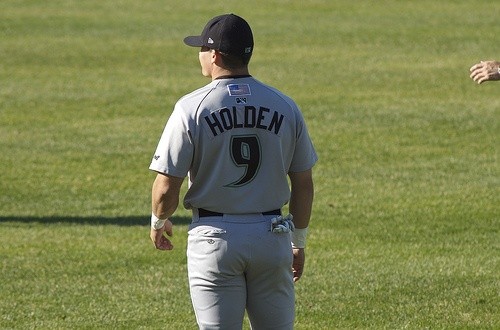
[199,208,281,217]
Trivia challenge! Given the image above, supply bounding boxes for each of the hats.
[183,13,253,53]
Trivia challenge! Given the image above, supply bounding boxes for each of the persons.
[148,14,318,330]
[470,60,500,84]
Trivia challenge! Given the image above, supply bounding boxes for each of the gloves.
[271,213,295,233]
